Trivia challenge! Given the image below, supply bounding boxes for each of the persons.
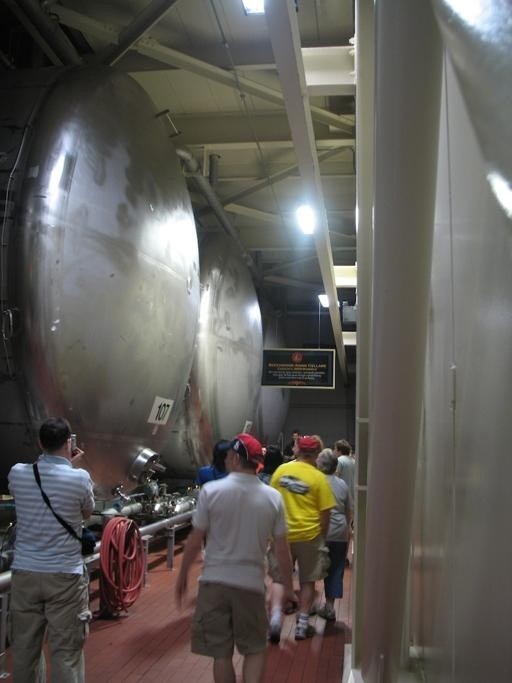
[7,416,96,683]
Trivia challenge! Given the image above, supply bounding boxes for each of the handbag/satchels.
[79,527,97,555]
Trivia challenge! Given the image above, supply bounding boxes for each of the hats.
[297,436,322,453]
[217,433,264,465]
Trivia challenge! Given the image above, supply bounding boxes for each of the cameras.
[71,433,77,450]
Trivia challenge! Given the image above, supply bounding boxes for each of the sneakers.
[269,616,283,643]
[317,605,336,621]
[295,624,317,639]
